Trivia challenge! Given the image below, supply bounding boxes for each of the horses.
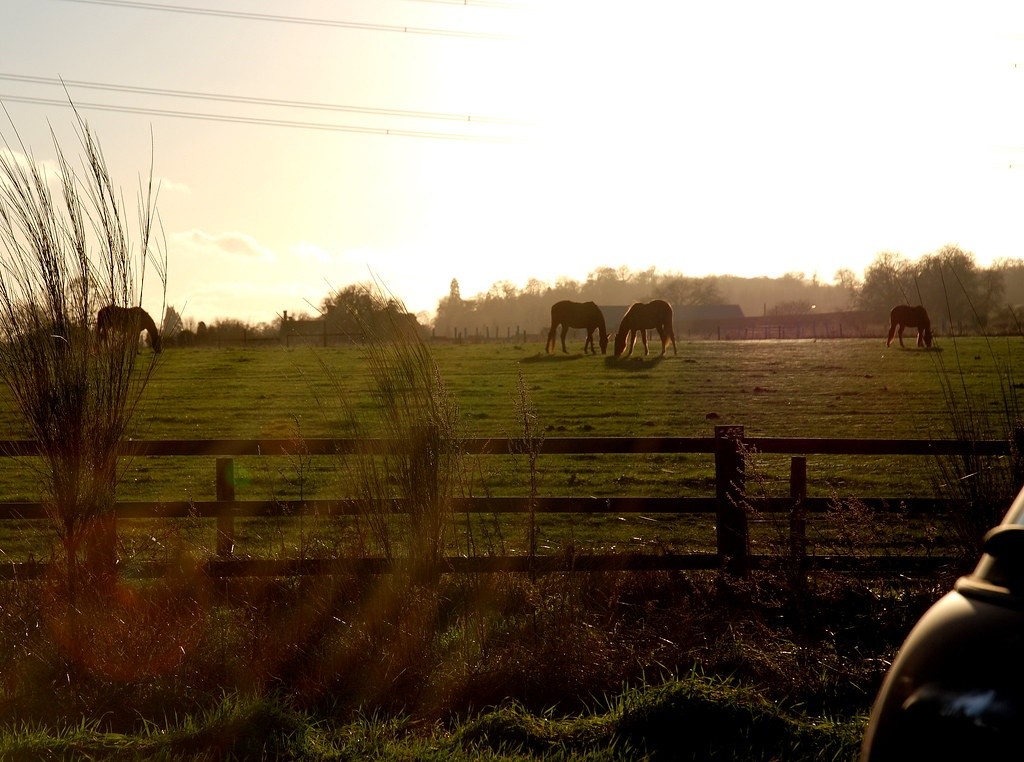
[545,300,611,356]
[92,304,163,355]
[614,300,677,358]
[886,305,933,349]
